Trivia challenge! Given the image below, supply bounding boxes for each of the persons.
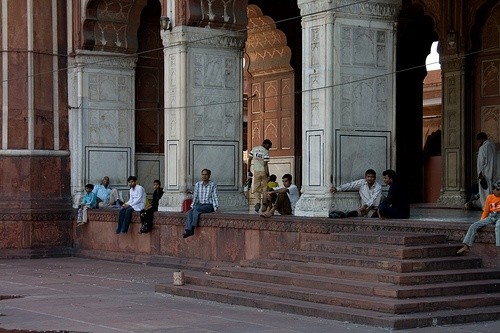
[140,180,164,233]
[379,169,410,219]
[260,174,299,218]
[330,169,383,218]
[476,132,496,213]
[116,176,146,234]
[77,184,96,226]
[183,168,219,238]
[267,174,279,188]
[457,179,500,253]
[248,139,272,212]
[92,175,121,208]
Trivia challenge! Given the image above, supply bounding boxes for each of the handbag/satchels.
[182,191,193,213]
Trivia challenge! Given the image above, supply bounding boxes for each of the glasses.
[282,180,288,182]
[493,186,500,191]
